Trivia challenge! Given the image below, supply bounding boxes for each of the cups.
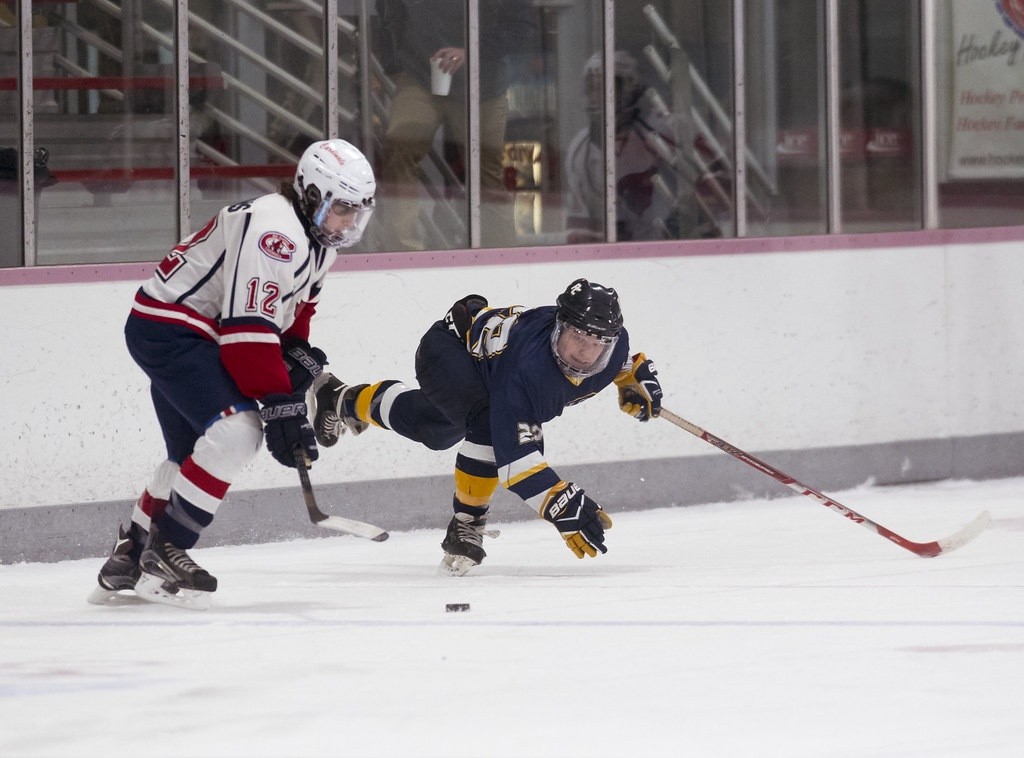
[429,58,452,96]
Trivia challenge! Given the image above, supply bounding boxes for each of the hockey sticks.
[296,448,389,544]
[623,392,992,560]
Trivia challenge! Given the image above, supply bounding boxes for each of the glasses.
[334,201,366,216]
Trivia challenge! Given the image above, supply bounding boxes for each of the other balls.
[446,602,470,613]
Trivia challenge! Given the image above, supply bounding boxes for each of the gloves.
[613,352,663,423]
[540,481,612,559]
[281,335,330,394]
[261,391,319,469]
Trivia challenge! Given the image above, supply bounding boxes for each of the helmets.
[292,138,376,207]
[580,53,645,129]
[556,278,624,343]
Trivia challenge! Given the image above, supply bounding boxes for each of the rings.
[452,57,459,62]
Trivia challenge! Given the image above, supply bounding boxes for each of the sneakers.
[86,524,180,606]
[439,512,500,578]
[133,511,217,611]
[307,372,371,448]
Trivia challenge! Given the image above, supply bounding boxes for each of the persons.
[313,280,663,565]
[376,0,522,253]
[98,139,376,592]
[563,51,733,244]
